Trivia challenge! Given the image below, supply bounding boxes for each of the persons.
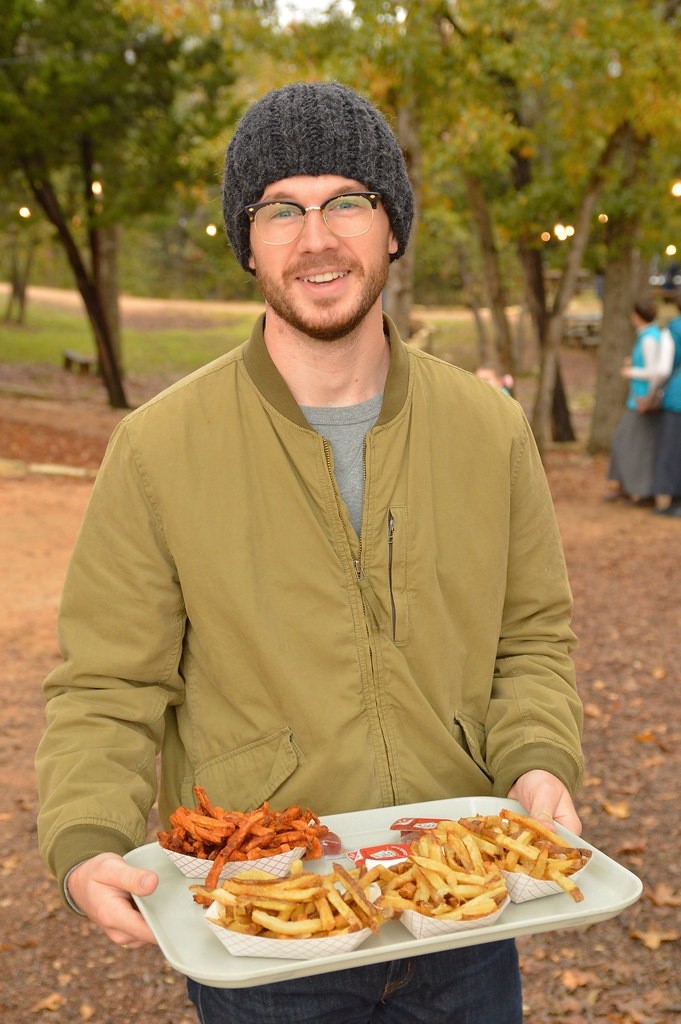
[603,287,681,515]
[33,84,583,1024]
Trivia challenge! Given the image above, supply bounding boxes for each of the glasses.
[244,191,382,245]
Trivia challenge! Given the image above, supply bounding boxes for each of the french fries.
[158,786,592,941]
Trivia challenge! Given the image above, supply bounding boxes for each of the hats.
[222,84,414,272]
